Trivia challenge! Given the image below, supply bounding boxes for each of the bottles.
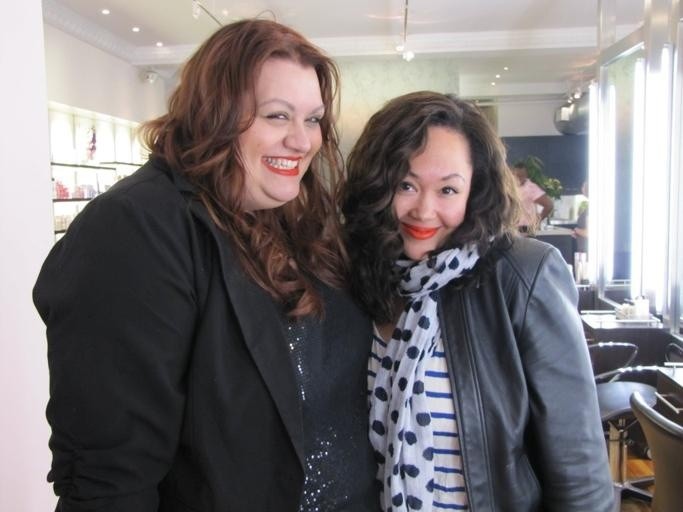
[50,173,96,232]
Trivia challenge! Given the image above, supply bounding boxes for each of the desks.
[581,313,671,385]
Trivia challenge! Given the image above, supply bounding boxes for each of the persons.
[33,5,379,512]
[570,180,589,254]
[511,161,553,237]
[336,91,615,512]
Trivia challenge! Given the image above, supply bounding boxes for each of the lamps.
[391,0,419,65]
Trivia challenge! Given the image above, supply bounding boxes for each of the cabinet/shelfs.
[49,158,115,235]
[655,366,682,425]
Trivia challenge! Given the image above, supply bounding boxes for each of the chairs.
[585,342,676,511]
[628,391,681,511]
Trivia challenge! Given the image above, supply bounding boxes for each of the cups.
[614,294,650,321]
[567,250,594,285]
[538,221,555,233]
[103,182,110,193]
